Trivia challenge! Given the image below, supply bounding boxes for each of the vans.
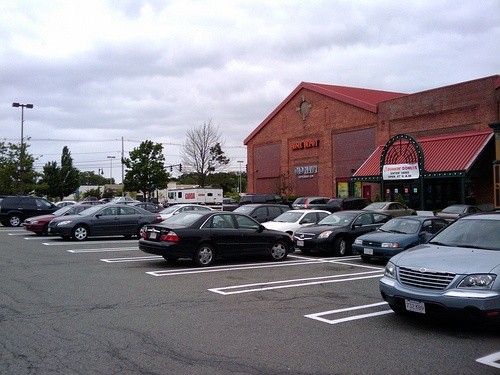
[0,195,61,227]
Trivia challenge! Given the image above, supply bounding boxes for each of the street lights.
[107,156,116,184]
[98,168,105,187]
[11,103,33,195]
[237,161,244,194]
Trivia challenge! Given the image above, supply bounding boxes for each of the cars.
[487,207,500,214]
[361,201,419,219]
[379,212,500,326]
[159,204,216,221]
[435,205,483,225]
[223,194,373,239]
[351,215,451,264]
[138,211,296,268]
[21,196,166,240]
[292,210,394,257]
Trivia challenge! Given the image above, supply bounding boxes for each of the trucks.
[167,188,224,210]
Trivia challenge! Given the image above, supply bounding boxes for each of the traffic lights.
[171,166,173,172]
[180,164,182,171]
